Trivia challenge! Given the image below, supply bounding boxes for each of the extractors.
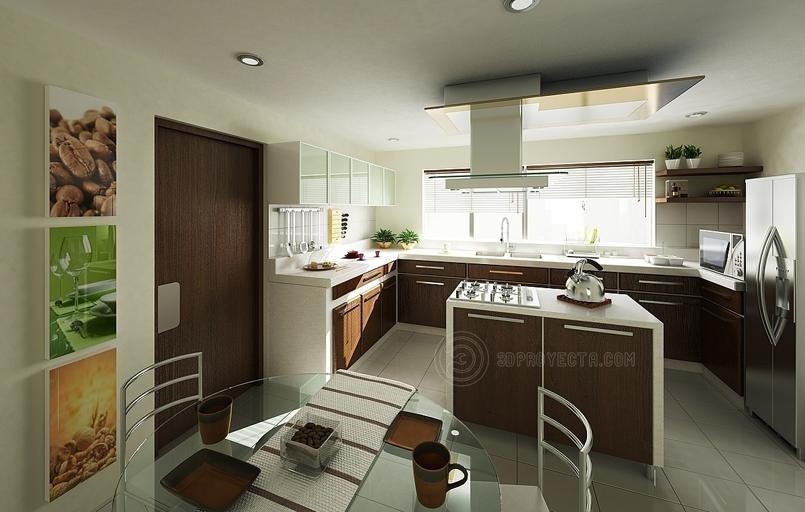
[443,73,549,191]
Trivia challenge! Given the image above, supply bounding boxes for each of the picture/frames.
[44,222,120,361]
[45,85,119,219]
[44,345,121,503]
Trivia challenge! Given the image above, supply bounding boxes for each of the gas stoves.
[448,280,542,311]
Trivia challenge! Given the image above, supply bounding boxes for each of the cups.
[375,251,380,258]
[358,253,364,260]
[198,394,234,445]
[412,441,468,509]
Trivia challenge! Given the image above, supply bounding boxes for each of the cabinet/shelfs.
[265,141,328,205]
[398,259,466,278]
[619,290,702,363]
[701,278,743,316]
[667,166,762,176]
[267,295,361,374]
[329,151,351,206]
[384,167,397,207]
[550,270,618,289]
[701,298,744,397]
[369,163,383,207]
[667,196,746,203]
[351,158,369,206]
[359,278,382,354]
[331,265,387,300]
[397,274,465,328]
[383,261,397,274]
[468,264,548,284]
[380,269,399,337]
[619,272,701,295]
[446,281,664,479]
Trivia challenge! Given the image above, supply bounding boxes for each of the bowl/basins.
[276,406,343,481]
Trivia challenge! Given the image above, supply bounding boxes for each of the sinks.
[475,250,542,259]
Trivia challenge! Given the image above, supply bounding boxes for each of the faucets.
[499,217,514,255]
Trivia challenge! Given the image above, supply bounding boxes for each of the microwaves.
[698,229,744,281]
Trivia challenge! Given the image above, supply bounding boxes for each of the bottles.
[671,186,679,197]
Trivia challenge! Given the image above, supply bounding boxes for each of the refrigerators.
[744,172,800,457]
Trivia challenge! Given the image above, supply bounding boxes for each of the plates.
[159,448,262,512]
[303,264,337,271]
[717,151,744,168]
[344,255,363,258]
[383,410,444,454]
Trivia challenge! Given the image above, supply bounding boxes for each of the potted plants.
[664,144,683,169]
[683,143,703,168]
[370,228,397,249]
[396,229,419,250]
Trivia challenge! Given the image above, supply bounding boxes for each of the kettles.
[565,257,605,303]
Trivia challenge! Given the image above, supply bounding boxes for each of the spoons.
[299,210,309,256]
[308,211,319,252]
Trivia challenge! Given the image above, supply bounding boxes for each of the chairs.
[500,385,594,512]
[120,351,203,490]
